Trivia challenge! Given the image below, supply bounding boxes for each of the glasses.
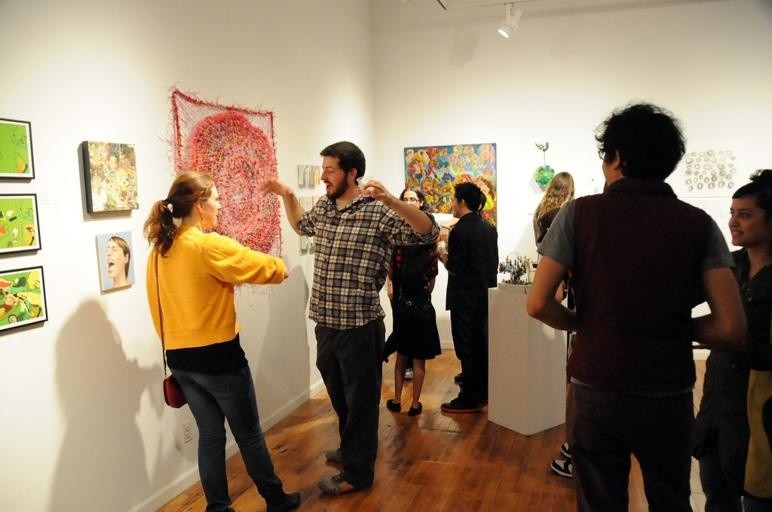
[404,198,421,205]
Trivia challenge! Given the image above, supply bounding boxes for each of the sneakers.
[408,401,423,415]
[265,492,301,510]
[441,401,481,413]
[549,458,577,478]
[561,441,574,457]
[405,368,412,380]
[324,447,345,460]
[317,473,366,494]
[387,398,401,413]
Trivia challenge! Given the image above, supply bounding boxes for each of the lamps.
[497,2,523,39]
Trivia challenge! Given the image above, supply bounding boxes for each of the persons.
[532,172,575,287]
[696,170,772,511]
[147,172,301,512]
[108,153,127,180]
[259,142,441,492]
[384,186,498,415]
[526,106,746,510]
[105,237,132,288]
[549,265,574,478]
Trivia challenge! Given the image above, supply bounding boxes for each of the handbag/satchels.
[162,373,189,408]
[743,367,771,501]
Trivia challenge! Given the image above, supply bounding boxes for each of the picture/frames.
[0,118,35,179]
[0,193,41,255]
[0,266,48,332]
